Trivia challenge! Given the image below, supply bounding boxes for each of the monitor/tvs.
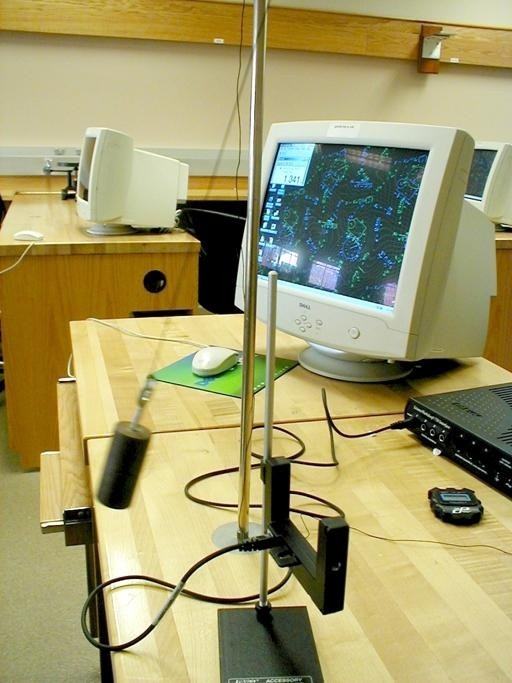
[233,119,497,386]
[464,141,512,233]
[75,126,189,236]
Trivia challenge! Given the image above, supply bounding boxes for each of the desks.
[39,313,512,683]
[0,193,202,472]
[481,228,512,373]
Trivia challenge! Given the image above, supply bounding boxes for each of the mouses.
[194,346,242,375]
[13,229,44,241]
